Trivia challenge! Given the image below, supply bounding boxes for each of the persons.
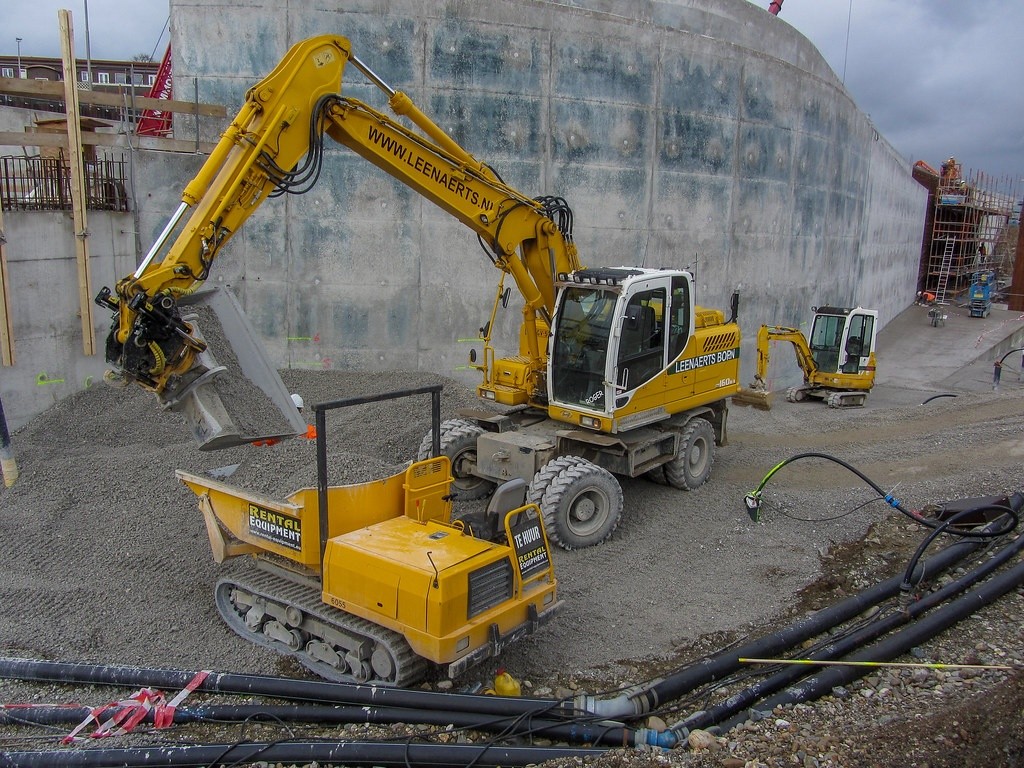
[979,242,987,264]
[916,291,936,307]
[948,156,966,196]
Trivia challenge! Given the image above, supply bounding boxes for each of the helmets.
[291,394,303,407]
[917,291,921,296]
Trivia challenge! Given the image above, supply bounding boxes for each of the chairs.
[581,304,655,389]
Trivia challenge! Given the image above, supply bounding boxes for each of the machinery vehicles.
[173,377,569,688]
[92,31,739,552]
[136,42,172,137]
[968,268,994,319]
[731,302,879,410]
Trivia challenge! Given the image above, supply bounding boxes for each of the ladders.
[935,235,956,305]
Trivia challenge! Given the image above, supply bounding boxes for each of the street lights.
[16,37,22,79]
